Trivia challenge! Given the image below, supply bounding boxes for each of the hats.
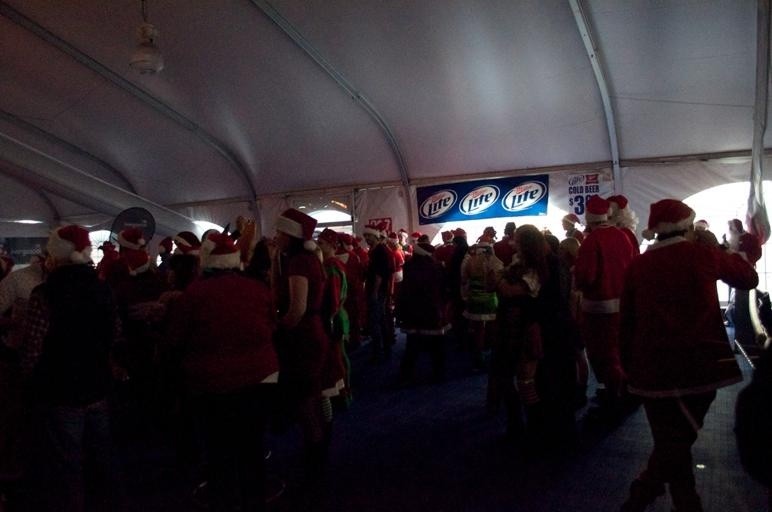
[178,232,202,248]
[127,249,150,271]
[603,193,629,210]
[729,218,746,233]
[440,230,452,242]
[411,231,422,241]
[158,236,173,250]
[362,223,379,236]
[202,229,221,238]
[338,232,358,245]
[277,207,318,252]
[199,237,241,269]
[380,224,398,242]
[478,236,492,245]
[485,228,495,235]
[47,224,93,265]
[585,195,609,222]
[397,228,409,238]
[322,228,336,249]
[454,228,468,238]
[695,220,712,228]
[415,244,435,257]
[118,231,145,247]
[641,199,696,241]
[563,213,581,226]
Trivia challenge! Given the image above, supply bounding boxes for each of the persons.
[2,195,772,511]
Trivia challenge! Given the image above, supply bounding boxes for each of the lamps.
[128,0,166,77]
